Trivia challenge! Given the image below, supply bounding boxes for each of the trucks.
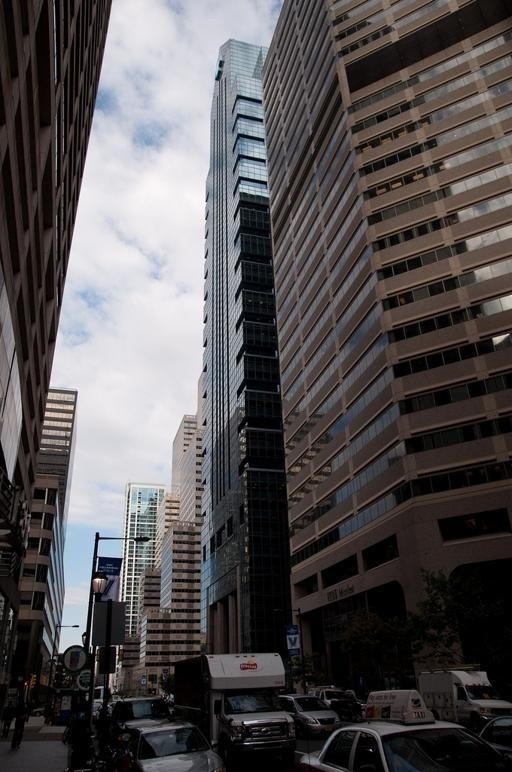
[175,653,296,772]
[418,669,512,726]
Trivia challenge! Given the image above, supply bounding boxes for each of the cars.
[279,685,512,772]
[31,706,48,717]
[93,686,228,772]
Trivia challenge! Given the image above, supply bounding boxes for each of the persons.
[0,701,26,751]
[62,694,98,772]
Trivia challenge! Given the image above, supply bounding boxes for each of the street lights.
[48,623,79,719]
[271,607,307,695]
[86,531,151,729]
